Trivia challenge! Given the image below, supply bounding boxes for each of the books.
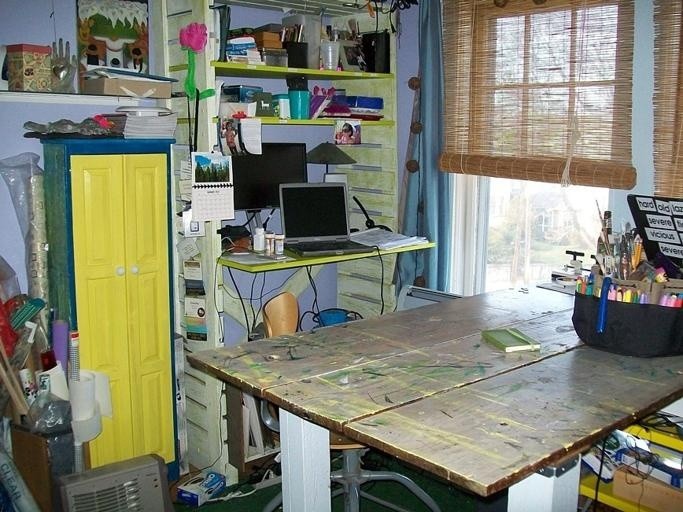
[349,228,427,250]
[101,112,177,141]
[584,427,682,488]
[481,327,543,354]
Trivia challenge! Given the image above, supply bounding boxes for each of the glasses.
[342,129,350,133]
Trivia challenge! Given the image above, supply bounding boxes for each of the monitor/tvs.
[229,143,308,211]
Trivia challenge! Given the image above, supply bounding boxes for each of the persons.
[220,121,240,155]
[334,121,359,143]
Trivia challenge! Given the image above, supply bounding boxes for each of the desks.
[188,280,683,511]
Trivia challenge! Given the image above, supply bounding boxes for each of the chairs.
[249,293,443,511]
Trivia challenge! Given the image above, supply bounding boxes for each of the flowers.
[178,21,213,100]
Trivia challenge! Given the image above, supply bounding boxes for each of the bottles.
[325,22,347,41]
[253,227,265,250]
[274,234,283,254]
[594,211,615,269]
[619,252,631,281]
[264,232,274,254]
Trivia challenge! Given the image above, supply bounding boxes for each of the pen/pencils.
[576,275,683,335]
[282,23,303,43]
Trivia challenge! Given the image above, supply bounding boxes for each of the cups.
[320,41,339,70]
[288,88,311,119]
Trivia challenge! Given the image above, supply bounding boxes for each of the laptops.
[279,181,374,259]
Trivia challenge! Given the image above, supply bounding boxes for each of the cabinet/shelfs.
[44,134,181,486]
[162,2,432,486]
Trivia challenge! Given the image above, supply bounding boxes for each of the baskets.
[591,265,682,292]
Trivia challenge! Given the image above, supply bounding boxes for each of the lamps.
[306,140,359,174]
